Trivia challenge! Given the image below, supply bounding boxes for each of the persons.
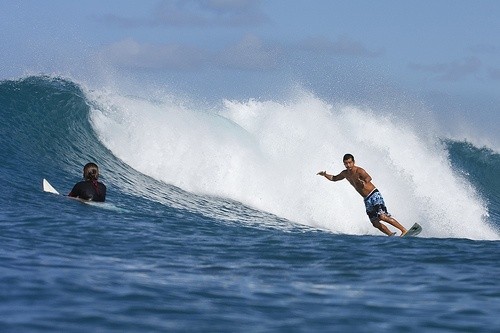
[316,154,407,236]
[68,163,106,202]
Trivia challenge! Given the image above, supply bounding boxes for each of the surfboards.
[402,221,423,238]
[43,179,59,194]
